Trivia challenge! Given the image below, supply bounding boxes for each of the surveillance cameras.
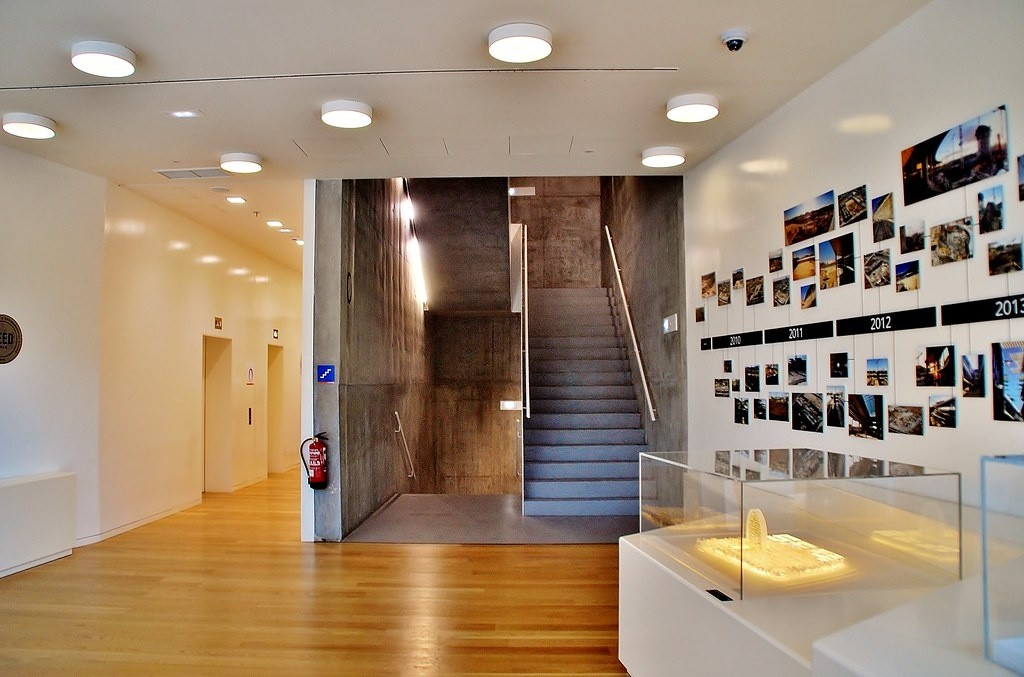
[720,30,747,51]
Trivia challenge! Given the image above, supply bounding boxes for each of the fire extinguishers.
[300,432,329,489]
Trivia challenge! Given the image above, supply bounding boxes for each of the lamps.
[71,40,137,77]
[642,146,687,168]
[2,112,57,140]
[220,152,263,174]
[489,23,554,63]
[321,100,373,128]
[666,93,720,123]
